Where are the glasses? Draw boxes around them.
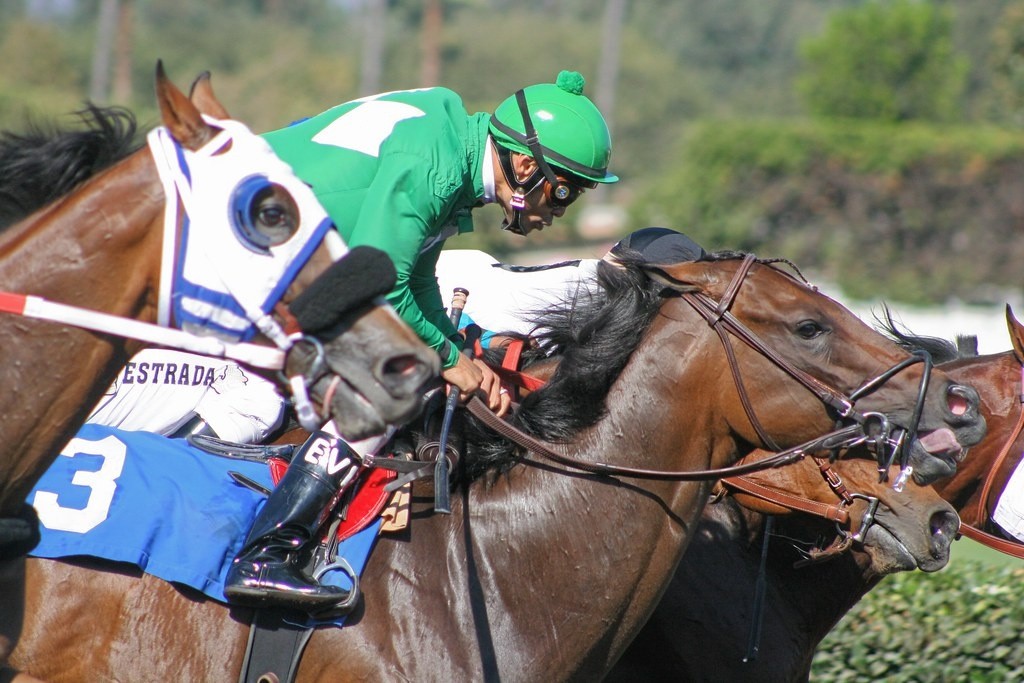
[514,89,584,209]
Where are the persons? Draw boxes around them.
[222,71,618,609]
[436,227,705,355]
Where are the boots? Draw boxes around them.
[222,429,351,616]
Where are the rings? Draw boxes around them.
[500,388,508,395]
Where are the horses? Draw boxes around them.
[0,55,1023,683]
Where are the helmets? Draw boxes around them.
[488,71,619,184]
